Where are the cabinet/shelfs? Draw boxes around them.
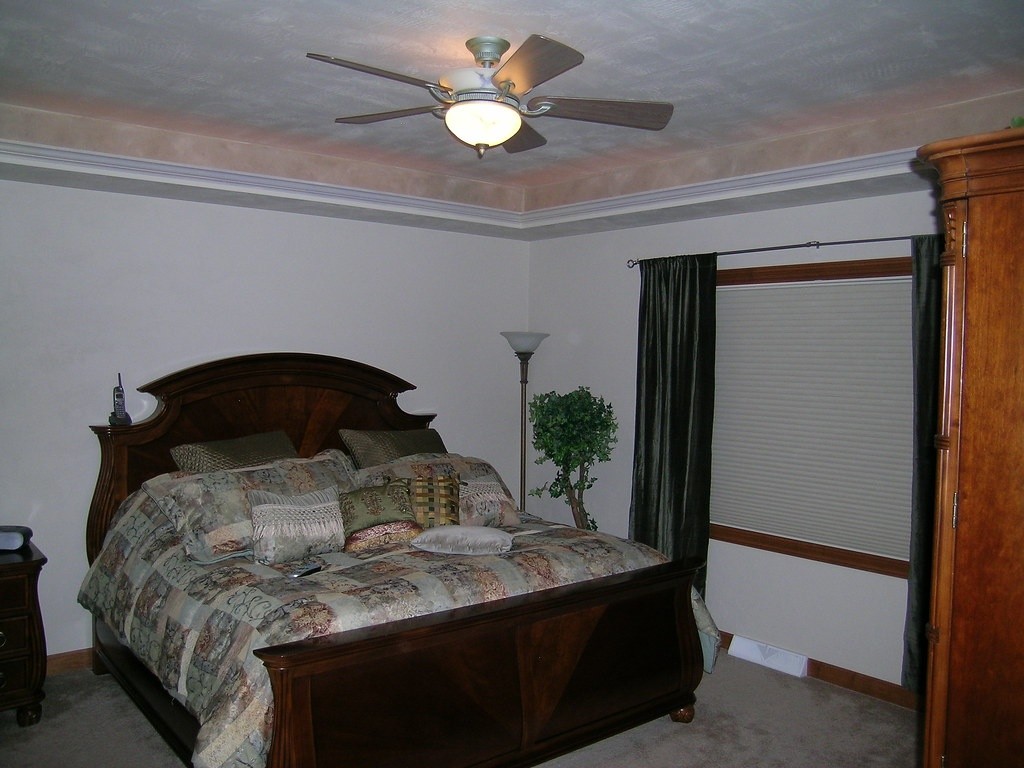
[0,527,47,728]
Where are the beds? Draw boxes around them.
[86,352,703,768]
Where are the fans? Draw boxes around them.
[306,35,673,160]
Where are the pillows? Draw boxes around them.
[350,453,523,528]
[170,429,300,476]
[246,485,344,566]
[141,446,362,564]
[338,478,418,539]
[411,526,515,554]
[338,428,449,470]
[409,473,461,530]
[346,520,423,550]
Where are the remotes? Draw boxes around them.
[288,563,322,578]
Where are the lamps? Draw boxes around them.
[499,330,550,510]
[445,88,522,160]
